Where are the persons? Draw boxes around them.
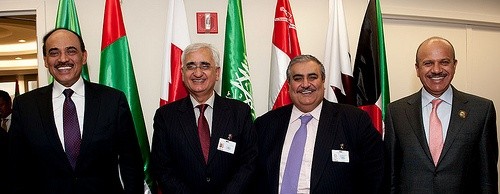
[254,55,383,194]
[7,28,144,194]
[148,43,258,194]
[384,37,499,194]
[0,90,12,133]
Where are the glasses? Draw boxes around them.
[181,64,215,71]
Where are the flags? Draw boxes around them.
[49,0,90,84]
[98,0,150,194]
[323,0,390,135]
[268,0,302,112]
[160,0,191,108]
[221,0,256,121]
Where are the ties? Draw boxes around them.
[429,100,444,166]
[195,103,210,165]
[280,114,313,194]
[62,89,80,172]
